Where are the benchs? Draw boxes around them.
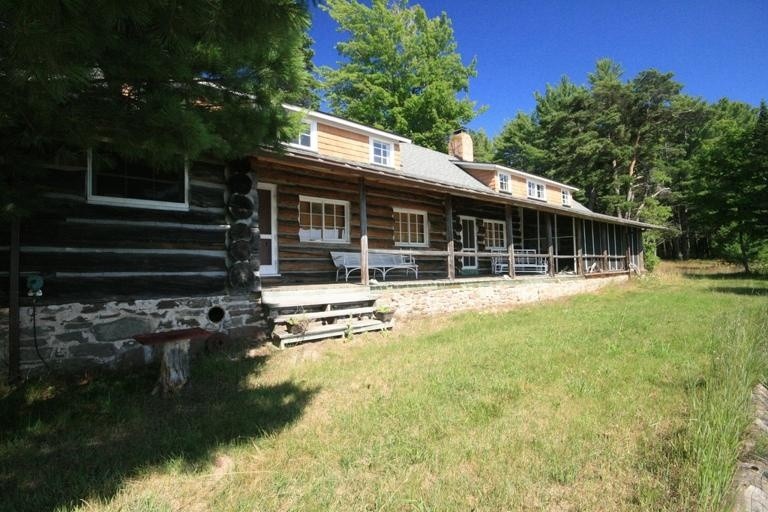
[491,247,548,274]
[330,251,419,283]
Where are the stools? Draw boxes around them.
[132,327,212,400]
